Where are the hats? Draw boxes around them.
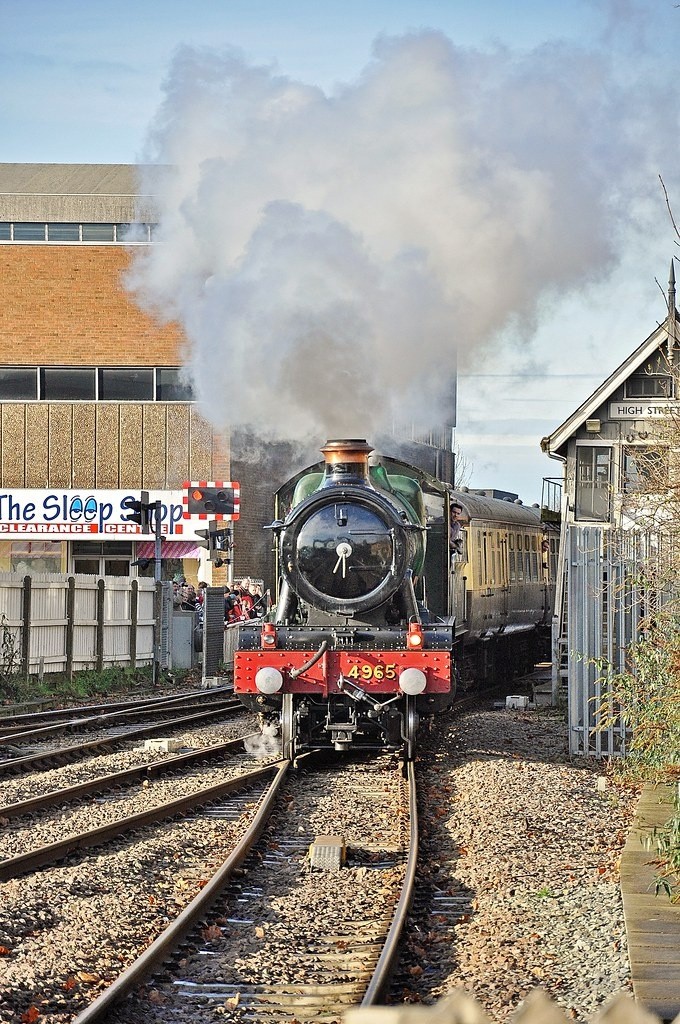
[222,585,230,593]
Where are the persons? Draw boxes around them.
[222,579,273,625]
[172,577,210,622]
[541,540,549,568]
[436,503,463,567]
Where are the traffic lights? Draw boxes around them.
[131,560,149,571]
[187,487,234,514]
[207,558,223,569]
[195,528,208,548]
[126,500,144,526]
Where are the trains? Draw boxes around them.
[231,437,568,763]
